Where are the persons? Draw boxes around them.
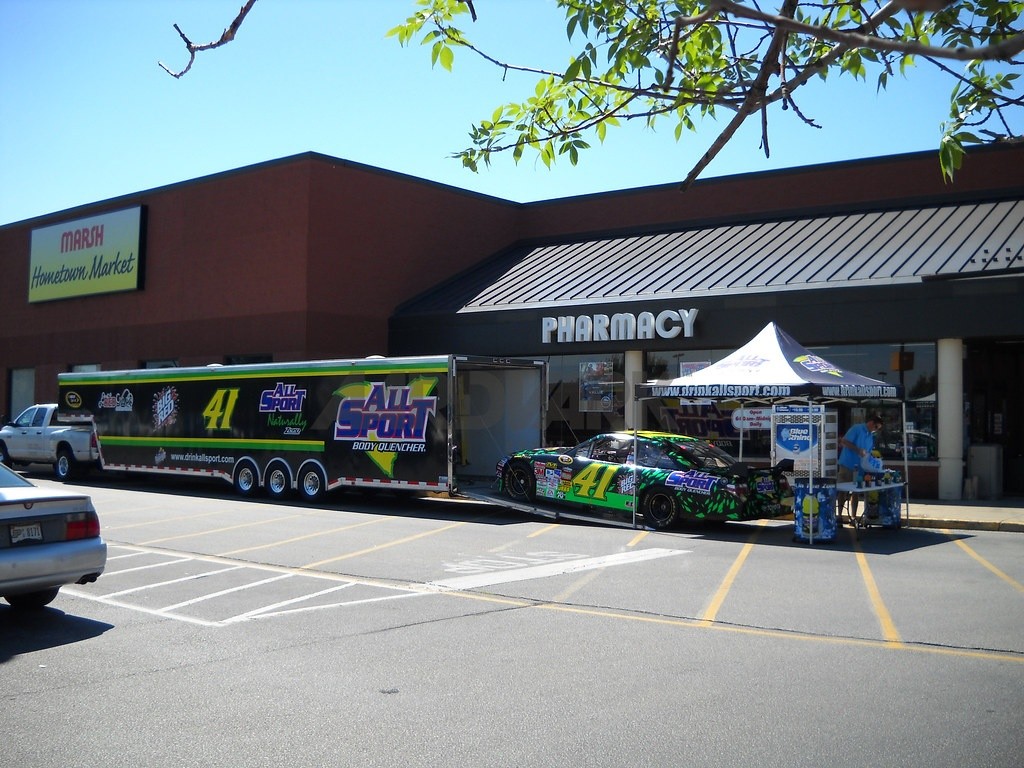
[836,416,883,527]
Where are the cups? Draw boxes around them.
[855,476,900,489]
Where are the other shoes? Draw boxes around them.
[849,519,861,526]
[836,519,842,528]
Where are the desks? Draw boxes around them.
[829,479,905,541]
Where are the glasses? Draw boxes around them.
[874,423,878,429]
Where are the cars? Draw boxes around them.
[497,427,795,532]
[0,464,108,609]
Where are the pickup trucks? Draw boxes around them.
[0,402,102,480]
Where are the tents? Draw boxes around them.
[632,322,909,543]
[908,393,935,408]
